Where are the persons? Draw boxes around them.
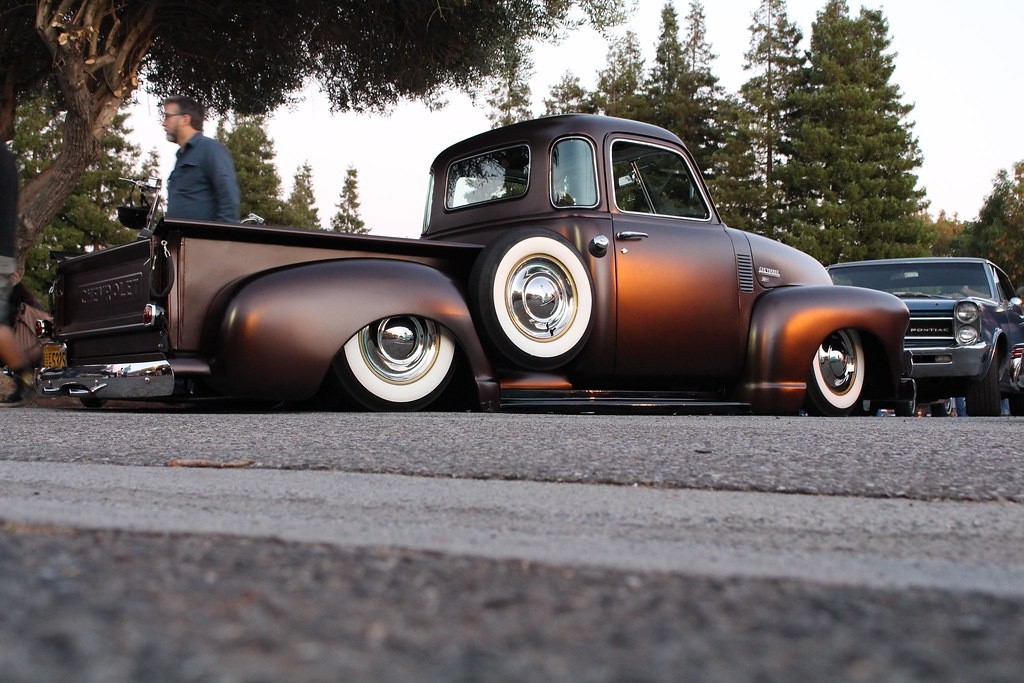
[877,396,1010,417]
[161,96,242,224]
[0,256,45,408]
[117,365,131,379]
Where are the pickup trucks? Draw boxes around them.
[31,113,919,417]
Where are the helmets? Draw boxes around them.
[116,202,153,229]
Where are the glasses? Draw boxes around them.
[164,113,185,119]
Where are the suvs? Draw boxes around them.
[826,256,1024,417]
